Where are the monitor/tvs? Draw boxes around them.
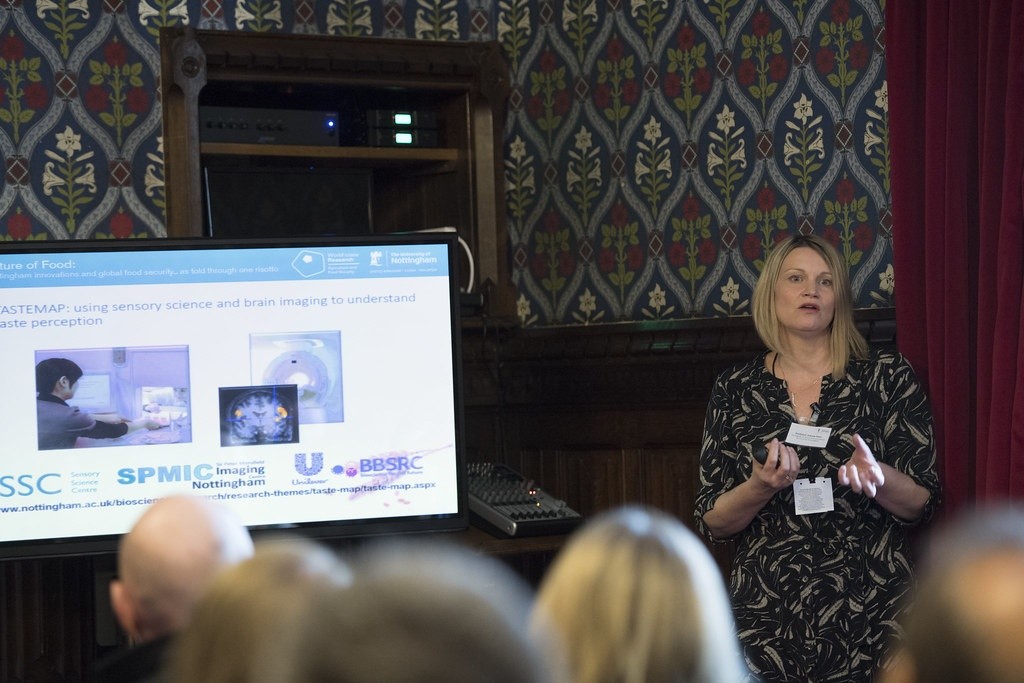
[0,230,466,561]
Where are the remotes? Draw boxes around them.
[752,442,780,469]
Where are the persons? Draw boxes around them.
[879,514,1024,682]
[695,235,942,682]
[35,357,164,452]
[533,506,741,683]
[109,496,552,683]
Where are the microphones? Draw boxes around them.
[810,402,822,413]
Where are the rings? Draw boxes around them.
[785,474,794,482]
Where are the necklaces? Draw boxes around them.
[778,354,832,407]
